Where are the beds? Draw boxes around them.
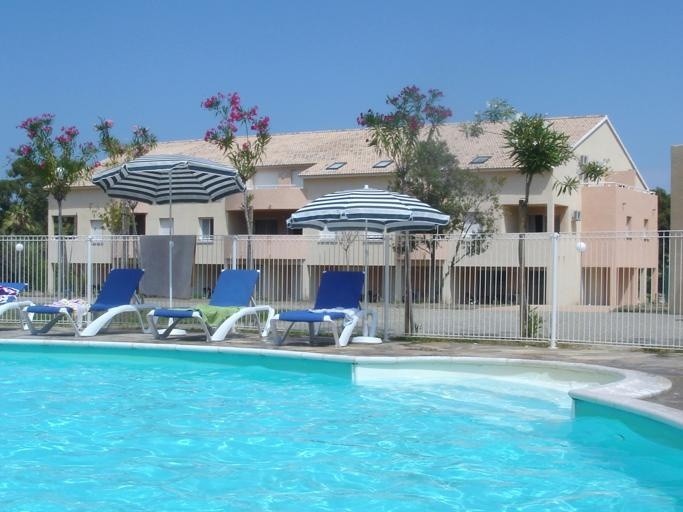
[283,185,451,337]
[91,153,246,307]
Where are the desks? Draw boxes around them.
[573,240,586,306]
[15,242,22,284]
